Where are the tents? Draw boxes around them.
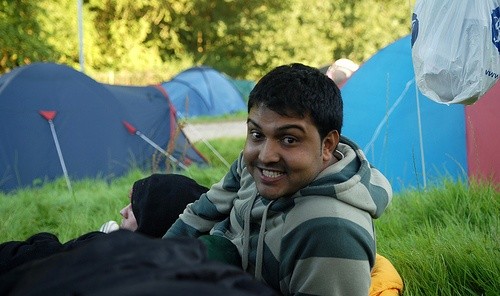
[0,63,245,193]
[340,36,500,197]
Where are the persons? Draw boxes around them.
[120,173,209,296]
[162,63,393,296]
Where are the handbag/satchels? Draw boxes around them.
[411,0,500,106]
